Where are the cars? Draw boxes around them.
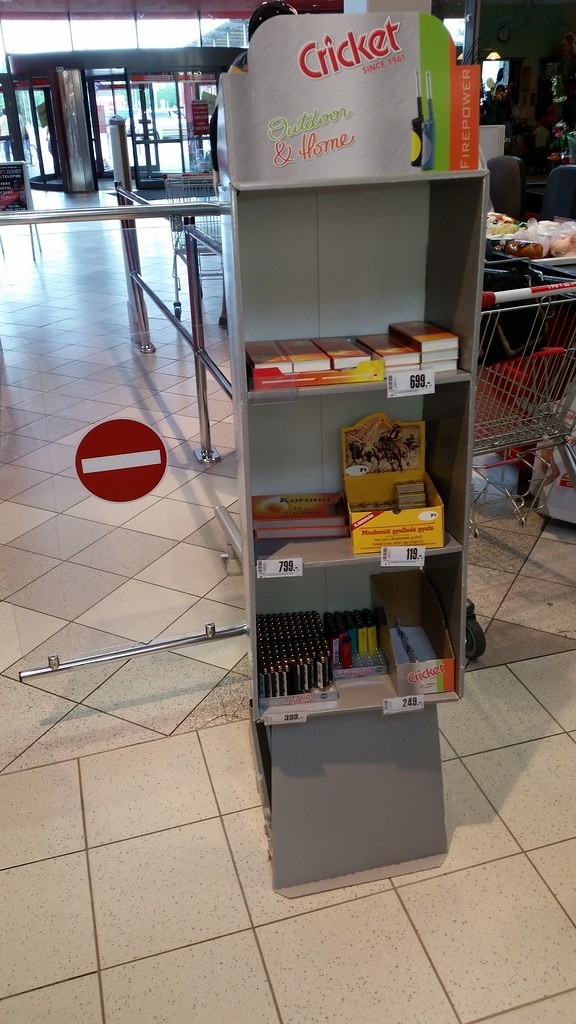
[123,105,211,147]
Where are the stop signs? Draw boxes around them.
[73,419,168,503]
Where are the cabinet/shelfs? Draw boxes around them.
[214,12,490,729]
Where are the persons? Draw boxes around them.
[496,85,513,139]
[0,107,32,162]
[531,103,558,170]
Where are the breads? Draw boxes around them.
[550,234,576,259]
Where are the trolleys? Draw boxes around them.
[471,281,576,538]
[162,172,223,321]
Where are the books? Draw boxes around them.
[245,321,459,377]
[251,493,349,539]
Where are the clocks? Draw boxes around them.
[496,26,511,43]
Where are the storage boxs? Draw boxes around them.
[251,492,345,528]
[332,650,388,679]
[368,570,456,697]
[258,681,340,708]
[256,527,349,539]
[340,413,446,555]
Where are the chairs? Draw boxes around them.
[540,164,576,223]
[486,154,527,222]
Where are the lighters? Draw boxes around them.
[256,608,377,698]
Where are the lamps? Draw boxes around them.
[486,50,501,60]
